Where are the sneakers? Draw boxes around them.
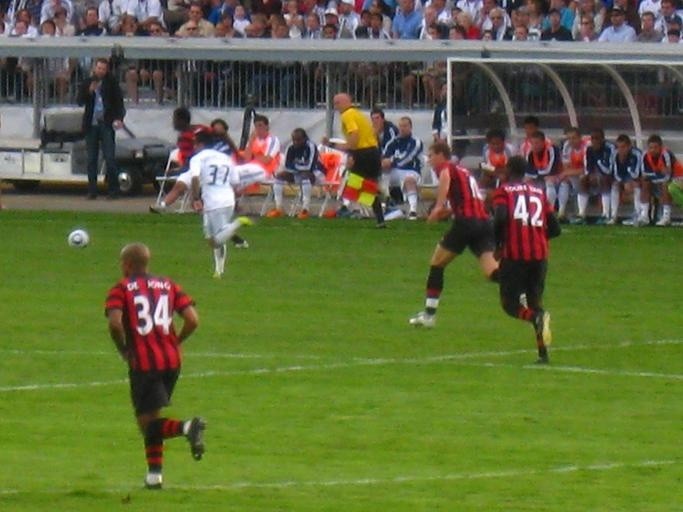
[529,358,550,365]
[408,312,435,328]
[142,480,161,489]
[534,311,551,348]
[299,210,307,218]
[211,272,220,279]
[558,215,671,226]
[264,208,281,217]
[106,191,119,198]
[406,211,416,221]
[185,417,204,461]
[83,192,96,199]
[148,201,165,215]
[236,215,251,227]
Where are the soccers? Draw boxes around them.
[66,229,90,247]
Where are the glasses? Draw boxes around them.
[150,29,158,33]
[489,16,500,20]
[580,21,589,24]
[185,27,197,30]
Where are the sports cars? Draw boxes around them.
[0,109,176,195]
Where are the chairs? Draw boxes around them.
[269,153,306,218]
[457,154,483,172]
[237,151,278,220]
[304,153,344,218]
[151,147,192,214]
[414,156,455,218]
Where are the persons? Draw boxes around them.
[408,142,528,328]
[0,0,682,121]
[104,242,206,489]
[77,58,126,201]
[321,93,386,227]
[484,155,560,365]
[151,109,281,215]
[265,108,682,225]
[188,131,253,280]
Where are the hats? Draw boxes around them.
[323,8,336,16]
[547,9,559,18]
[607,5,622,15]
[665,15,680,24]
[340,0,355,6]
